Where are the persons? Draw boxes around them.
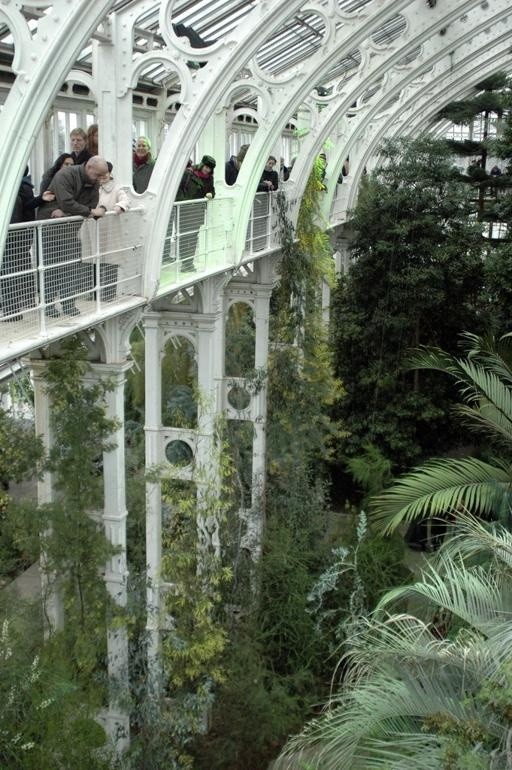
[132,136,155,194]
[226,144,251,185]
[0,124,128,322]
[337,156,350,184]
[162,155,216,273]
[319,154,328,179]
[253,156,289,254]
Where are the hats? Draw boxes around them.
[199,155,216,174]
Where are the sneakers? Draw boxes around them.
[45,306,81,317]
